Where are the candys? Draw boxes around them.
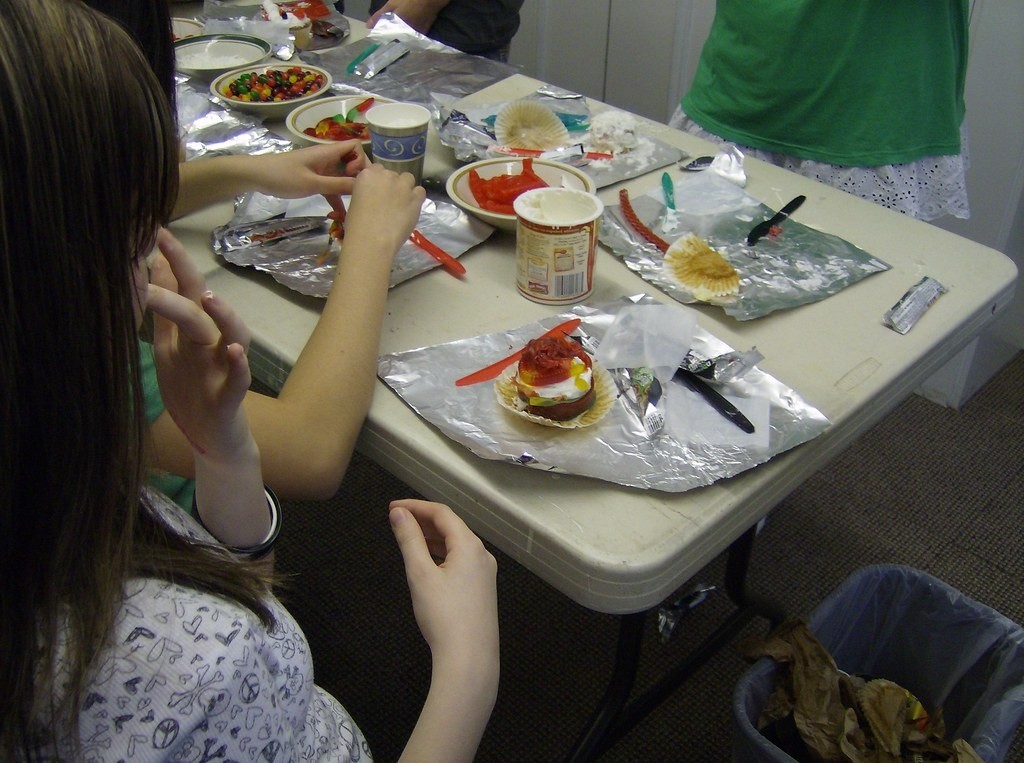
[304,97,376,142]
[222,66,324,103]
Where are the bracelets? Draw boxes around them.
[190,480,283,562]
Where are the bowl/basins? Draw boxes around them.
[446,157,597,234]
[171,17,205,41]
[174,33,272,81]
[285,95,402,156]
[210,63,333,121]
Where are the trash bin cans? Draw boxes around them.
[731,563,1024,763]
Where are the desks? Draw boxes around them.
[150,13,1018,763]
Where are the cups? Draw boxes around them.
[513,187,604,306]
[368,102,431,187]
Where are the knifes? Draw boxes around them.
[747,195,807,246]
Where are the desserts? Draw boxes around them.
[270,7,313,49]
[515,336,598,421]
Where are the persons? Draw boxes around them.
[368,0,525,65]
[667,0,971,534]
[82,0,426,526]
[0,0,499,763]
